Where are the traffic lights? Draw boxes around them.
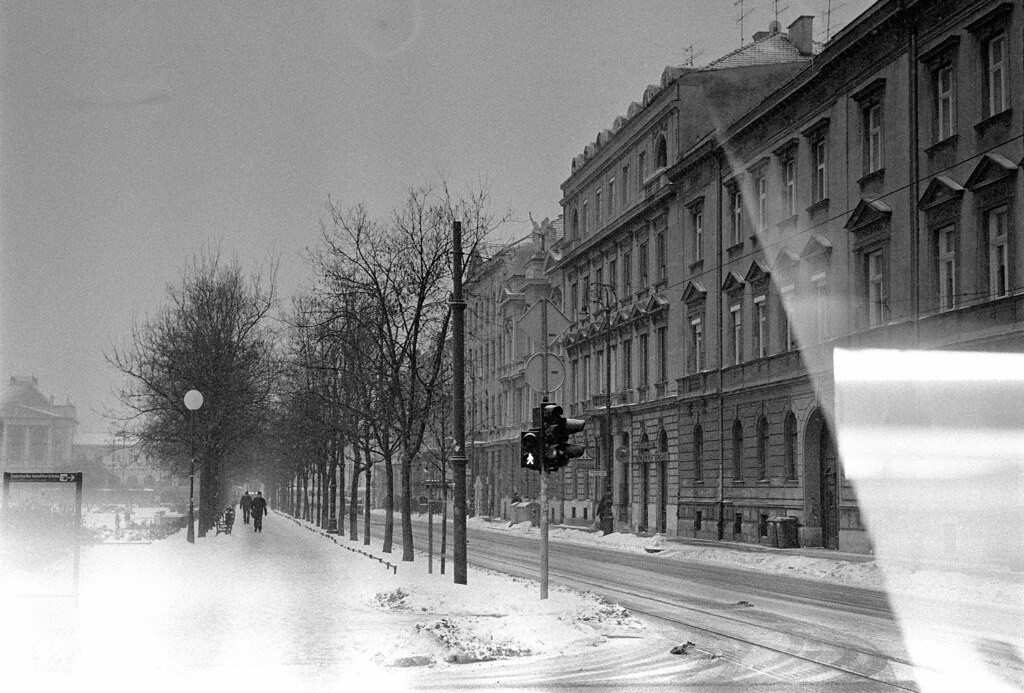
[562,418,586,465]
[520,431,540,470]
[541,402,565,466]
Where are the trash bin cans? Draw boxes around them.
[766,516,800,549]
[419,503,429,516]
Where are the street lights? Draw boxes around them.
[579,282,613,536]
[183,390,204,544]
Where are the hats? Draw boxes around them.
[245,491,248,494]
[257,492,261,495]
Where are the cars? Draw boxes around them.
[343,496,363,514]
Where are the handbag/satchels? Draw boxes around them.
[251,509,257,517]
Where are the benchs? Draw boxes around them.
[214,511,232,536]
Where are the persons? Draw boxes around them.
[419,494,427,503]
[251,491,267,532]
[512,492,523,503]
[540,219,548,251]
[240,491,251,524]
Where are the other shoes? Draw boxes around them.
[255,527,256,531]
[259,528,262,532]
